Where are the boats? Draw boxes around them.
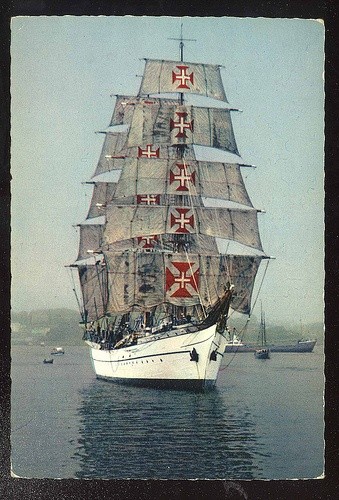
[44,359,53,363]
[51,348,65,355]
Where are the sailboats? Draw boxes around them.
[63,34,277,392]
[220,299,319,353]
[255,299,270,360]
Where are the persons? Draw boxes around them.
[86,322,124,350]
[157,313,191,333]
